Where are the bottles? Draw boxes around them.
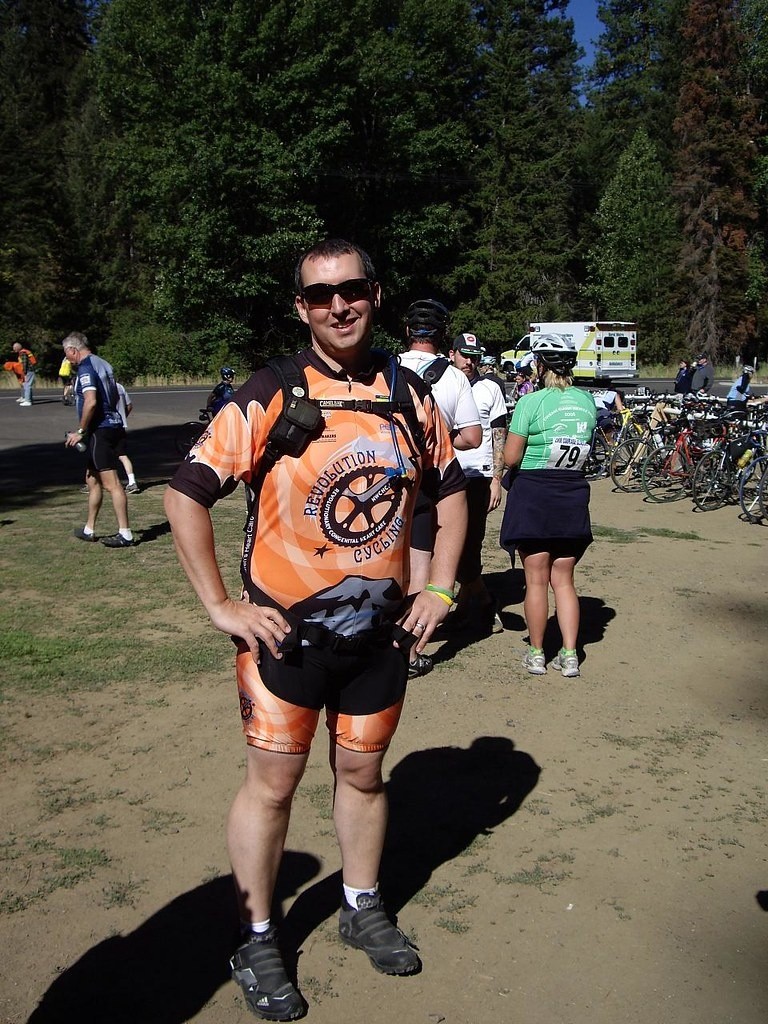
[65,430,86,452]
[654,433,665,453]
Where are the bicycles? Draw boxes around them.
[175,408,213,457]
[63,377,76,406]
[584,391,768,524]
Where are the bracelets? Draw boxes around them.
[424,582,460,612]
[76,428,86,436]
[493,466,503,481]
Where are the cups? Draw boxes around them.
[737,449,753,467]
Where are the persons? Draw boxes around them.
[726,366,755,420]
[165,240,468,1021]
[391,298,483,678]
[504,333,598,677]
[4,342,37,406]
[478,355,534,403]
[61,332,135,547]
[207,368,236,419]
[80,382,140,494]
[592,389,624,475]
[58,357,77,403]
[447,333,507,634]
[675,354,715,396]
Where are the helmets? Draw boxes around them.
[742,365,756,375]
[403,299,451,338]
[531,332,580,375]
[220,367,237,376]
[480,355,498,367]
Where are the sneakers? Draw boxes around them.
[100,533,136,548]
[227,926,309,1023]
[408,652,434,679]
[521,651,548,675]
[71,526,97,542]
[551,648,581,678]
[338,895,418,973]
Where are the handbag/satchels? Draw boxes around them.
[705,418,729,437]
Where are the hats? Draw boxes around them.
[452,333,484,355]
[695,352,708,360]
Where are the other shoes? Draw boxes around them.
[468,596,503,632]
[59,396,72,406]
[124,483,141,494]
[81,481,89,494]
[15,397,32,406]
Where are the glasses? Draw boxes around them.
[298,278,373,304]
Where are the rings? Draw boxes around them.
[416,623,425,631]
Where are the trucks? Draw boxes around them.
[500,321,639,388]
